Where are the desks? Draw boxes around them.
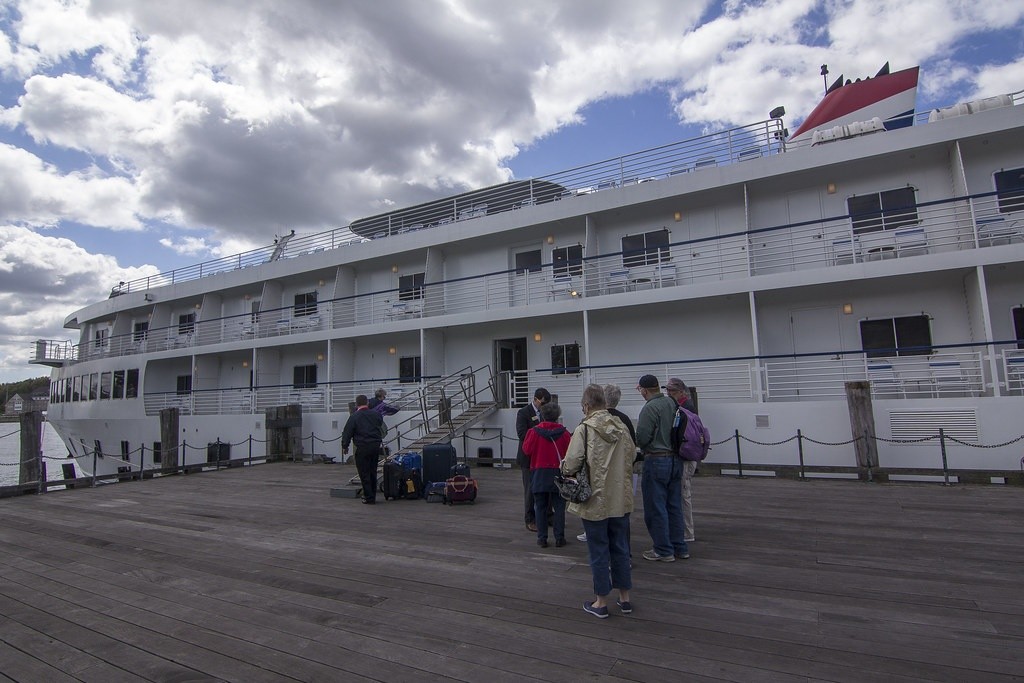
[903,379,934,398]
[631,279,651,292]
[867,246,896,262]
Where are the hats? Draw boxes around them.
[635,374,659,389]
[661,377,686,391]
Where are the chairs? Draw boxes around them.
[207,144,762,279]
[384,384,403,407]
[174,331,192,349]
[603,261,677,295]
[832,226,931,266]
[278,315,320,336]
[546,273,573,302]
[383,300,406,322]
[122,338,143,356]
[976,217,1024,246]
[867,355,974,399]
[1009,351,1024,396]
[289,390,323,413]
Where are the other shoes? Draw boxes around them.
[555,538,566,547]
[583,601,609,619]
[362,499,375,505]
[617,597,632,613]
[537,538,548,548]
[684,536,696,542]
[361,495,367,500]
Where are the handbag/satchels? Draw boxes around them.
[553,422,591,504]
[424,480,447,503]
[378,420,388,439]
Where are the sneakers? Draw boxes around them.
[576,532,587,542]
[674,547,691,559]
[640,549,676,562]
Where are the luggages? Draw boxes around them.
[395,451,422,472]
[381,439,403,501]
[422,442,458,482]
[451,462,471,477]
[444,474,478,505]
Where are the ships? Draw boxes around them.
[28,87,1024,483]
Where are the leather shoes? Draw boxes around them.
[526,521,538,532]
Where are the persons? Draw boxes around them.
[576,385,634,569]
[522,403,570,547]
[659,378,698,543]
[516,388,553,530]
[559,384,633,619]
[634,375,690,563]
[342,388,402,503]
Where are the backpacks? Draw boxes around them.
[401,467,424,500]
[667,394,711,461]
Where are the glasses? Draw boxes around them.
[383,396,386,398]
[534,396,545,406]
